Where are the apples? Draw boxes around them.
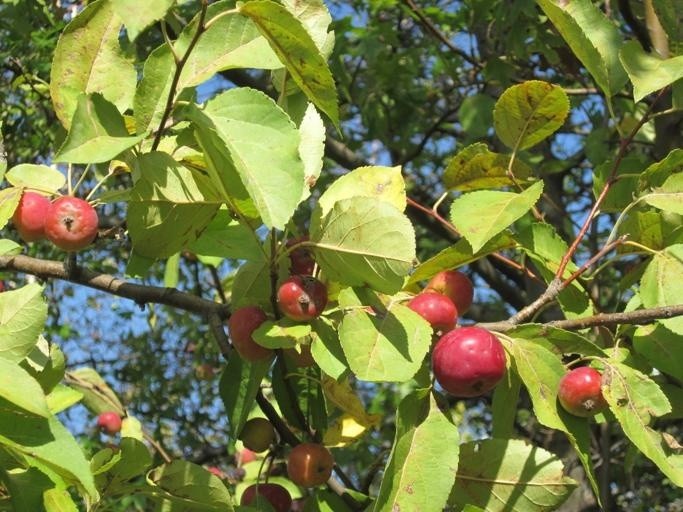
[11,191,607,512]
